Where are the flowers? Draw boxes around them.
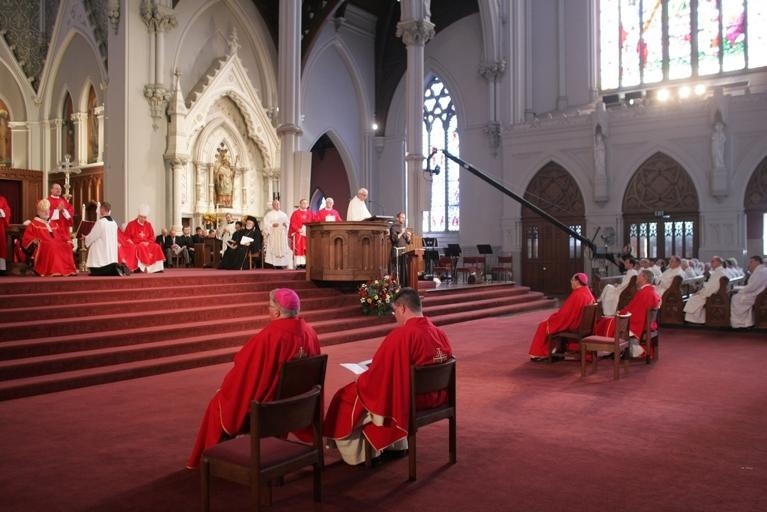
[356,271,402,317]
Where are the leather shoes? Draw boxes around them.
[371,450,408,465]
[168,264,172,268]
[115,263,130,276]
[603,352,623,360]
[531,357,549,363]
[186,264,191,268]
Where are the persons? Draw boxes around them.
[617,245,635,274]
[287,199,319,268]
[389,213,411,288]
[323,286,453,466]
[595,255,767,330]
[347,187,372,221]
[215,156,236,208]
[317,197,341,221]
[262,199,290,267]
[0,183,262,276]
[528,272,595,361]
[183,289,325,471]
[595,270,659,360]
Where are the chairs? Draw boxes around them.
[624,309,659,365]
[592,275,767,333]
[200,385,321,510]
[428,255,514,286]
[580,313,632,381]
[548,303,601,364]
[364,355,457,480]
[266,355,329,486]
[157,238,265,271]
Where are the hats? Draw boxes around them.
[137,205,150,217]
[275,289,301,309]
[579,273,588,284]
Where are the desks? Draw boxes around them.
[5,223,37,274]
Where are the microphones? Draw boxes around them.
[368,200,385,214]
